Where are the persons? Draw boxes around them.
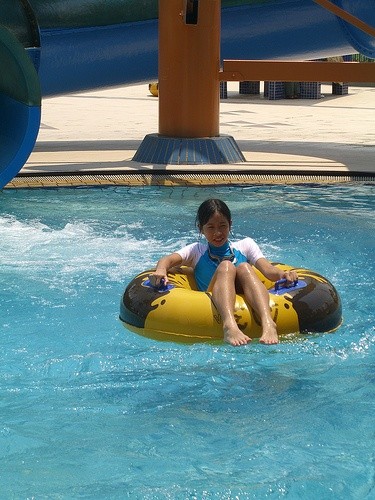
[148,198,298,347]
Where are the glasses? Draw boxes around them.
[208,242,235,264]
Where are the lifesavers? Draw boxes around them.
[91,366,317,421]
[118,260,345,347]
